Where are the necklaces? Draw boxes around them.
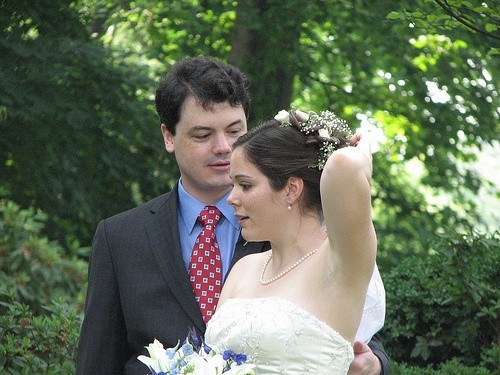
[260,249,320,283]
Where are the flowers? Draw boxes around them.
[136,326,257,375]
[274,107,355,172]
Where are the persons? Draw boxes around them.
[196,110,388,375]
[69,54,397,375]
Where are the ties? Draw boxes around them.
[188,206,224,326]
